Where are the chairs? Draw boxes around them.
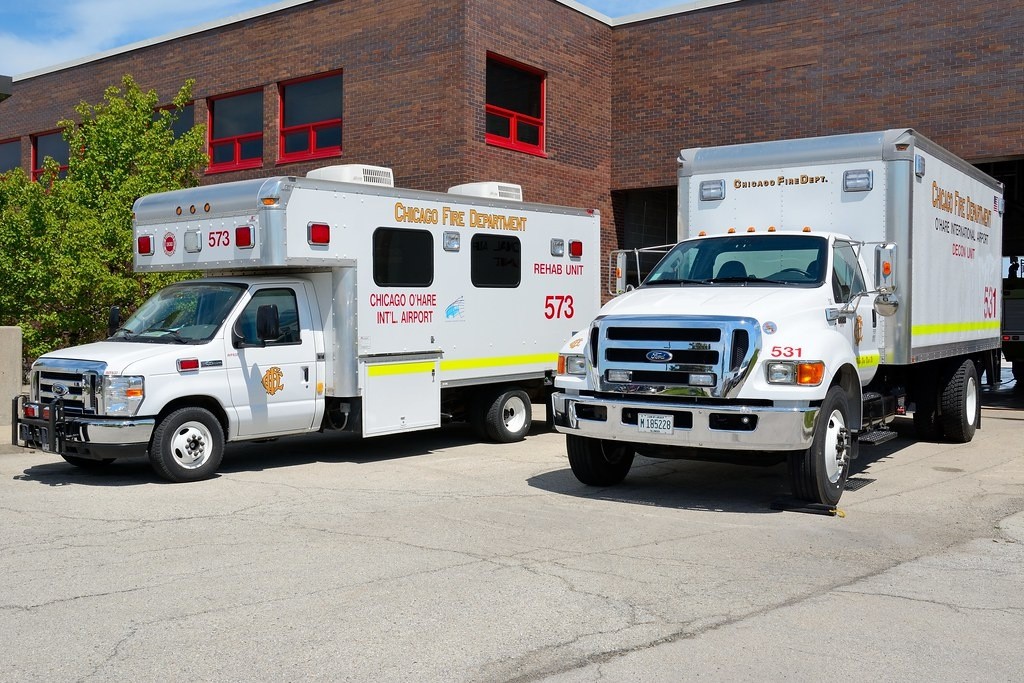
[256,305,280,344]
[807,260,843,302]
[715,260,747,280]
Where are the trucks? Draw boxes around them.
[551,127,1002,510]
[11,164,603,485]
